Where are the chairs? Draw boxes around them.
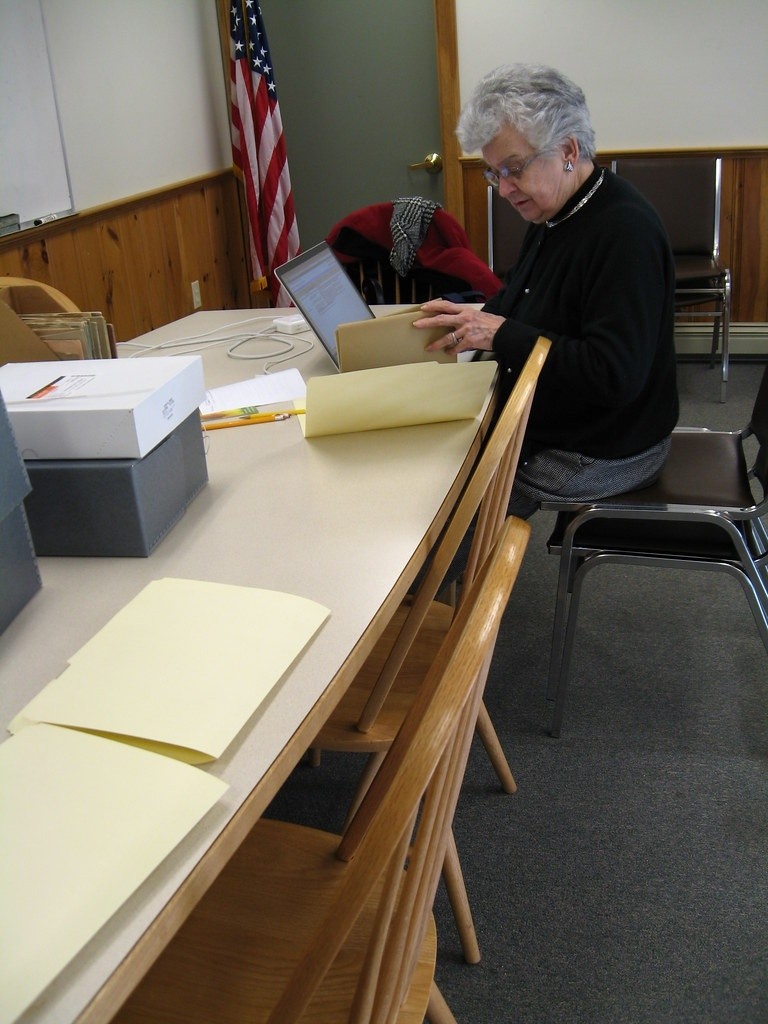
[487,184,529,287]
[111,514,531,1024]
[306,336,554,966]
[609,156,731,403]
[322,199,465,310]
[538,368,768,740]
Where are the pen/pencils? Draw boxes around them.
[199,406,306,430]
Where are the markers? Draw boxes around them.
[34,214,57,226]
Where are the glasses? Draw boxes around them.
[482,150,545,187]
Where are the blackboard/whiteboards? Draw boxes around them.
[0,0,235,257]
[433,0,768,173]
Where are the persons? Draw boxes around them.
[405,60,683,600]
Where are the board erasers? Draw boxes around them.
[0,212,22,236]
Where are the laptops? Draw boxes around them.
[274,241,376,372]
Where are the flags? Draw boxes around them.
[228,0,305,310]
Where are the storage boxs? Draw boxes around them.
[0,354,206,460]
[21,406,211,558]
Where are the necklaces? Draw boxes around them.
[543,169,606,229]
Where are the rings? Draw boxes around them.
[451,332,459,346]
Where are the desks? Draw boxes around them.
[0,302,511,1024]
